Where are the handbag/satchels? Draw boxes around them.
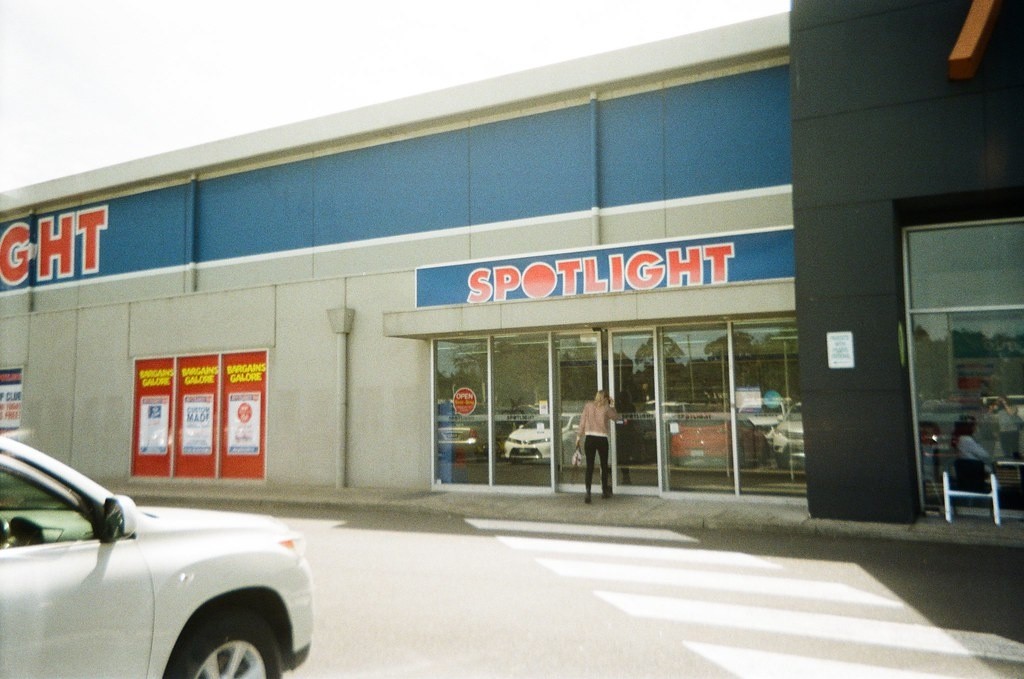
[571,445,582,467]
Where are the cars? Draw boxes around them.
[739,396,795,441]
[773,401,805,471]
[503,413,609,468]
[631,400,695,443]
[666,412,770,469]
[984,395,1024,438]
[0,435,313,679]
[435,423,513,463]
[916,398,984,457]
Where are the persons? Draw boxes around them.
[988,393,1020,460]
[946,416,993,494]
[619,390,646,464]
[576,390,622,503]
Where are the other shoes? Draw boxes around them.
[584,495,591,503]
[602,491,611,498]
